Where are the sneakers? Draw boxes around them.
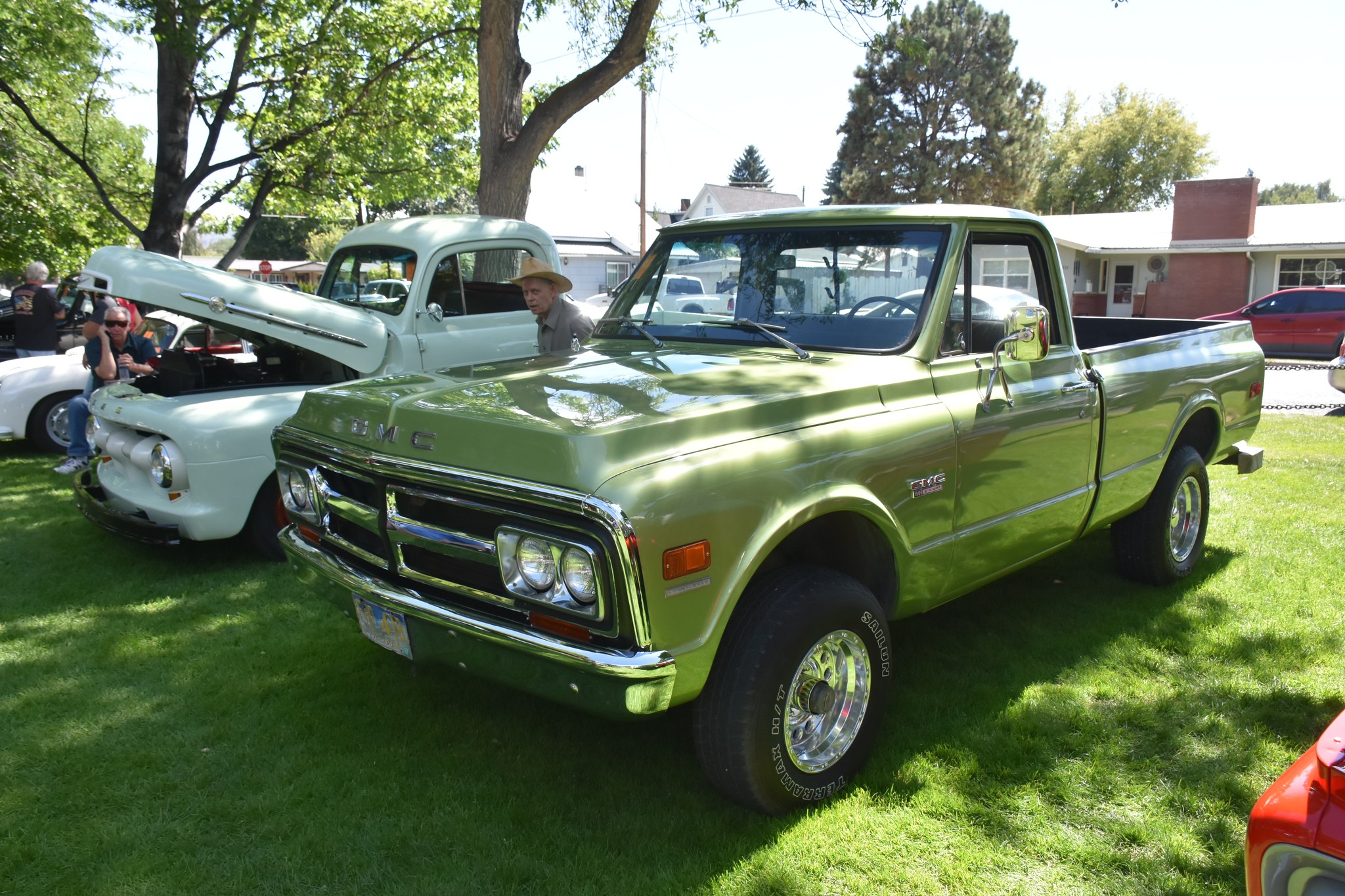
[52,457,92,474]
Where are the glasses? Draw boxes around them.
[103,319,128,329]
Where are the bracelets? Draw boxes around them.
[150,368,155,374]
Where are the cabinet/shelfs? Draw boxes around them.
[1073,292,1122,317]
[1132,293,1146,318]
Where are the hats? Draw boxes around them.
[504,257,573,294]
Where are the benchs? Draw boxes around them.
[773,314,1005,351]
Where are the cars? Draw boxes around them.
[0,269,163,362]
[832,284,1041,321]
[1193,286,1345,360]
[267,278,412,305]
[73,212,665,563]
[0,309,259,463]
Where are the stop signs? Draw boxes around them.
[259,261,272,275]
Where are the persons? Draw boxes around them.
[83,290,118,340]
[505,255,594,355]
[114,296,142,333]
[11,262,64,358]
[53,307,158,474]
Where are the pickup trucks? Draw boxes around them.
[269,202,1268,824]
[584,274,737,318]
